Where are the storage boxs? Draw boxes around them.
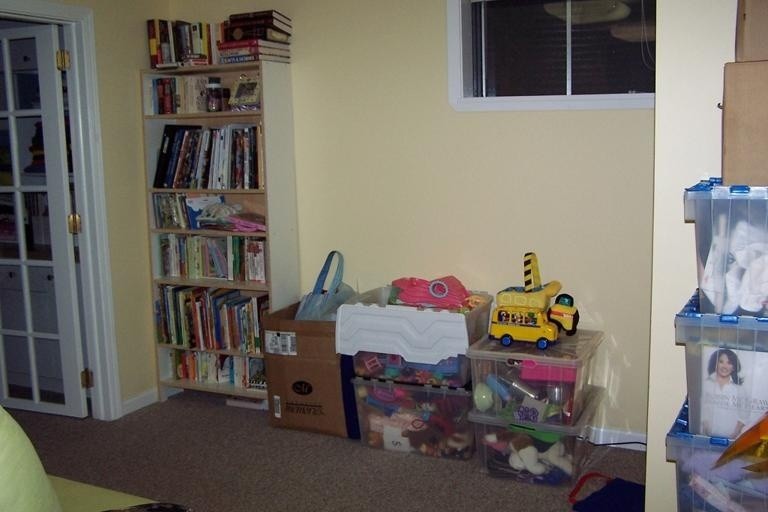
[468,324,604,426]
[261,291,355,436]
[345,287,494,390]
[722,59,767,187]
[345,375,477,461]
[670,290,767,447]
[679,174,768,320]
[663,395,767,512]
[734,0,767,63]
[484,383,609,486]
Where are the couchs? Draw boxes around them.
[0,402,160,512]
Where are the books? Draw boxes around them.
[152,282,266,356]
[152,124,265,190]
[169,347,266,392]
[156,234,265,282]
[152,191,264,232]
[146,9,292,67]
[151,76,209,114]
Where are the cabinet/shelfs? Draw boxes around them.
[0,38,88,398]
[139,61,302,404]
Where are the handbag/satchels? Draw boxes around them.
[295,251,353,321]
[569,472,645,512]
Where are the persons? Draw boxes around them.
[701,349,749,439]
